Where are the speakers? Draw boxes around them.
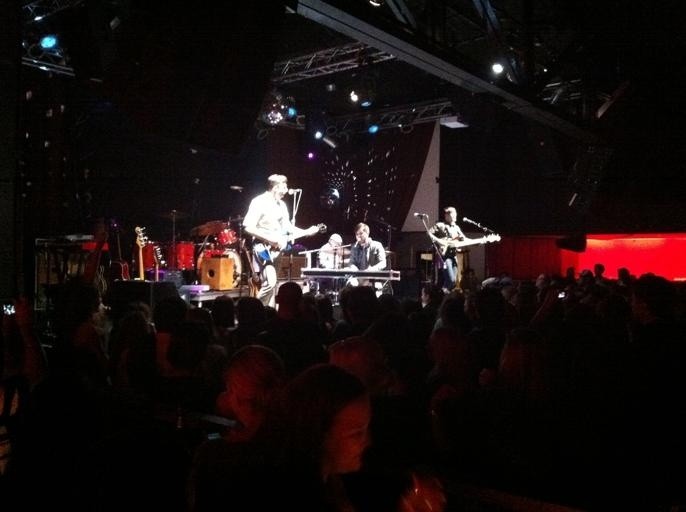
[576,208,614,233]
[112,278,190,329]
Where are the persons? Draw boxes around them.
[316,232,344,268]
[242,173,319,311]
[2,263,686,512]
[346,222,387,298]
[426,205,468,293]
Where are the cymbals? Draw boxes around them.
[190,219,233,244]
[159,209,190,221]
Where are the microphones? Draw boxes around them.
[413,212,427,218]
[462,217,482,228]
[354,237,362,248]
[288,188,301,195]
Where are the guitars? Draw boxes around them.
[429,234,502,259]
[251,223,328,266]
[108,219,131,282]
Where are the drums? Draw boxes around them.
[167,241,198,272]
[212,228,239,248]
[196,246,245,289]
[130,240,156,273]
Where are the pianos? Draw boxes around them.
[301,267,402,282]
